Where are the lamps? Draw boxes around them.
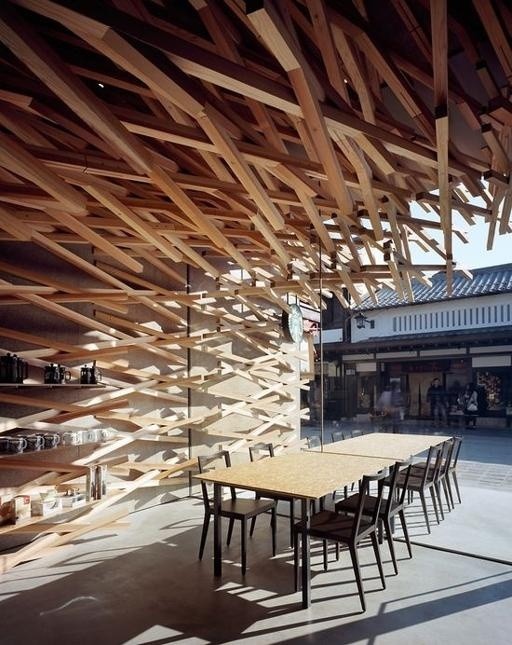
[353,309,375,330]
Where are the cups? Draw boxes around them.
[0,352,103,385]
[0,428,112,455]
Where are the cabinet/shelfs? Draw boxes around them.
[0,380,123,541]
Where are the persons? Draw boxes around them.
[307,376,480,431]
